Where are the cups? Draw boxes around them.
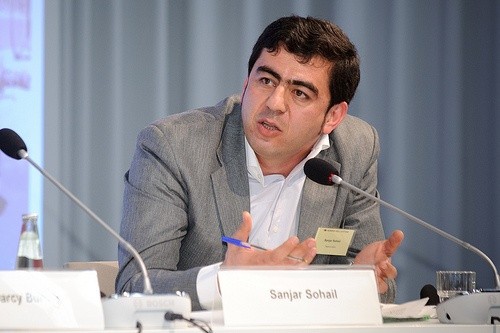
[436,271,477,303]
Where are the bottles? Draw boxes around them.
[16,214,43,271]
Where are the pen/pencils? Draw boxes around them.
[221,236,309,264]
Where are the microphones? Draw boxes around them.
[304,158,500,325]
[0,128,192,330]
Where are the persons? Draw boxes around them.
[113,14,404,313]
